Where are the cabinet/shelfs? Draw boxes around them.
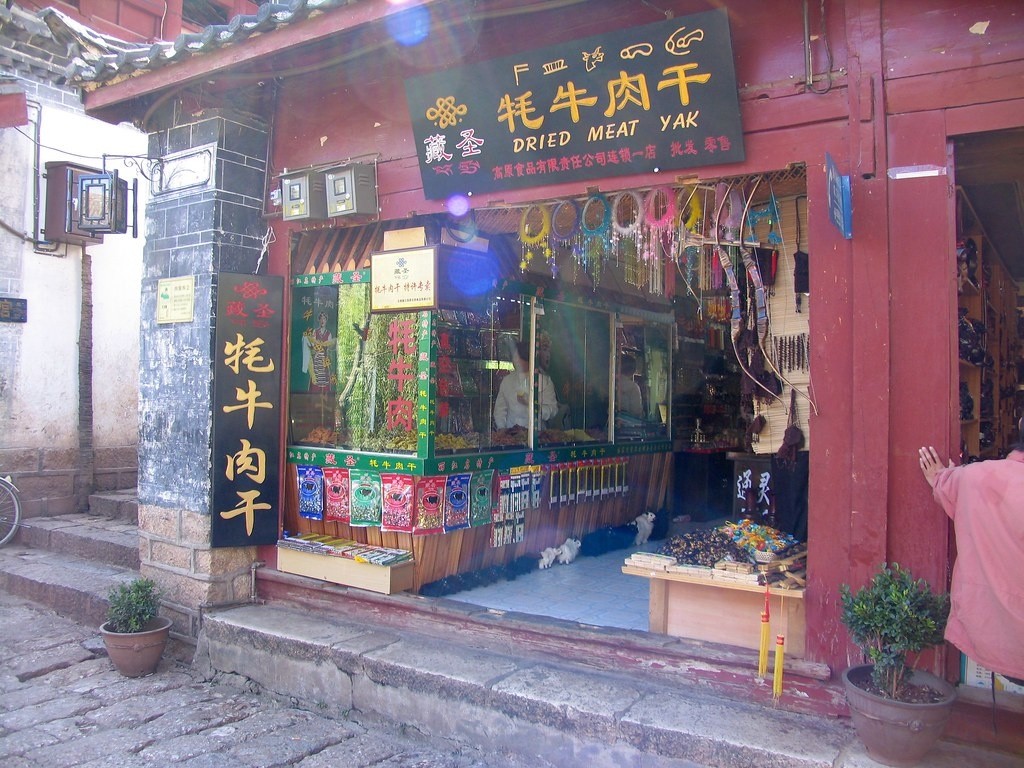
[277,548,417,594]
[953,184,1019,466]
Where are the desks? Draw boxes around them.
[724,450,810,543]
[669,445,744,523]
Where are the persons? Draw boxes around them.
[618,354,644,422]
[494,341,558,430]
[918,410,1024,698]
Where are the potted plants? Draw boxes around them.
[835,559,956,768]
[99,570,175,677]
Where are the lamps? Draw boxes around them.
[887,165,946,179]
[60,155,164,239]
